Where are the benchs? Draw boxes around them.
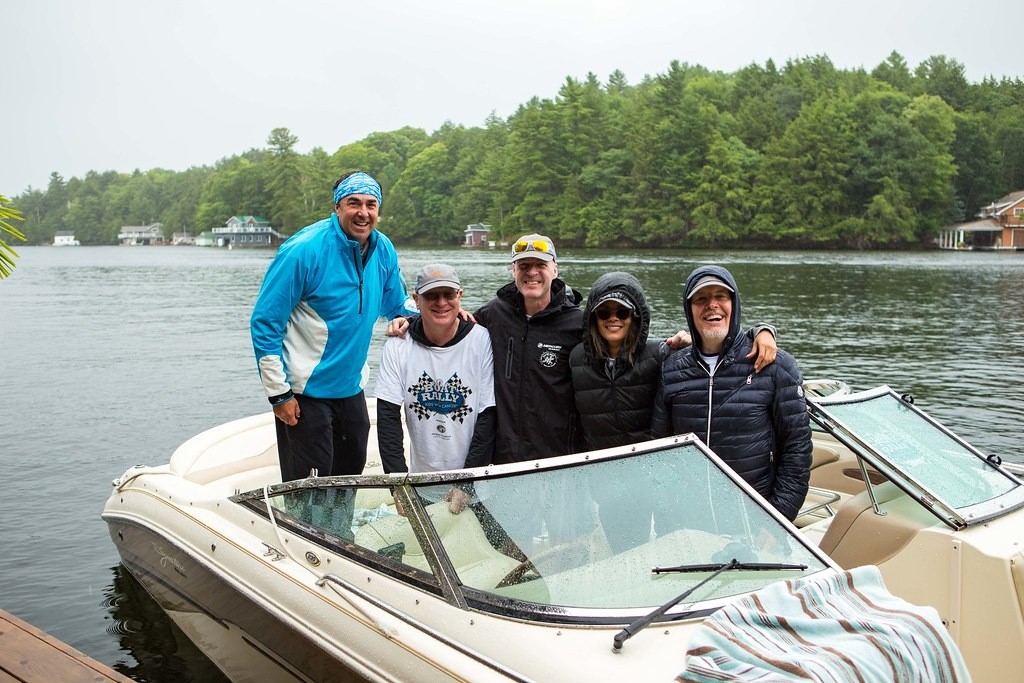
[792,439,890,527]
[169,397,412,515]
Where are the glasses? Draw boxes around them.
[512,240,554,258]
[422,290,459,300]
[594,308,631,320]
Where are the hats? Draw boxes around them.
[591,292,633,312]
[510,233,556,263]
[687,275,734,299]
[414,264,460,294]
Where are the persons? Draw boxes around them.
[652,265,813,557]
[390,232,695,564]
[250,169,478,540]
[375,263,497,515]
[570,270,778,452]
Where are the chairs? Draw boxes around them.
[354,501,535,591]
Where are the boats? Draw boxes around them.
[102,383,1023,683]
